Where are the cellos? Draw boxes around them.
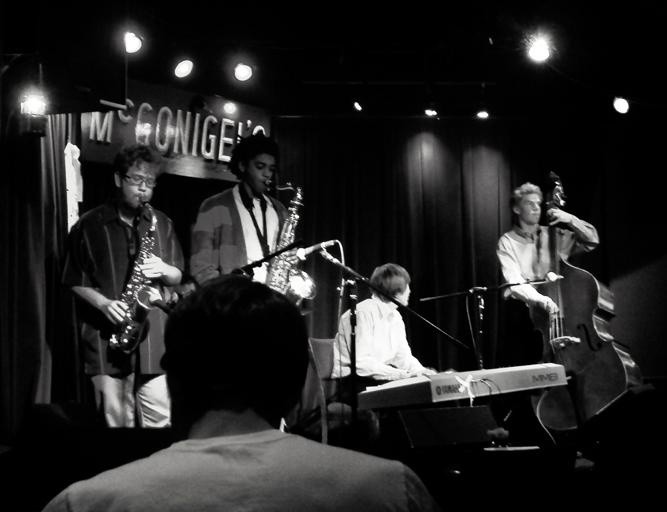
[529,172,644,438]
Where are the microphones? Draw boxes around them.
[148,294,170,317]
[546,271,564,284]
[295,238,335,260]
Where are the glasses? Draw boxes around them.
[124,174,156,188]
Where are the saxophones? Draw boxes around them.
[109,199,167,354]
[264,178,318,308]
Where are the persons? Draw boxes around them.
[42,142,186,433]
[328,261,443,448]
[489,180,601,452]
[41,272,443,511]
[188,131,302,315]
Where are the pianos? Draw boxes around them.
[358,363,569,411]
